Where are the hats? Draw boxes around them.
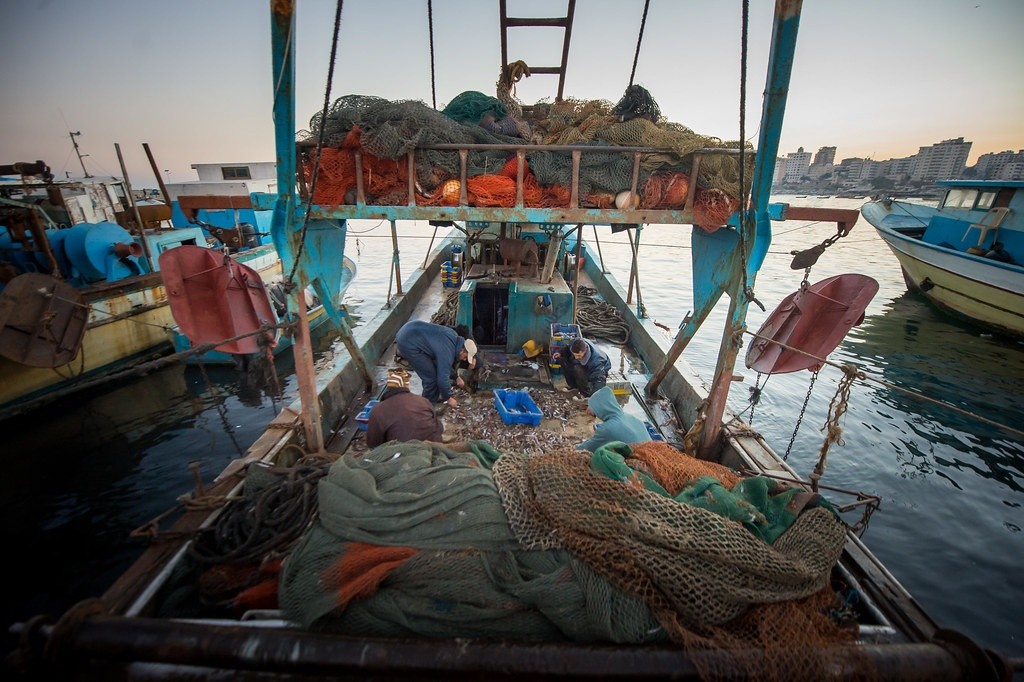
[464,339,477,364]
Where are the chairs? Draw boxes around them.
[961,207,1011,246]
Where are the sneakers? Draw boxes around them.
[562,386,578,392]
[573,393,589,401]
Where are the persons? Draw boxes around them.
[552,337,611,417]
[571,386,652,453]
[396,319,483,409]
[367,387,444,450]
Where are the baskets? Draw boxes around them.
[440,261,461,287]
[355,400,381,431]
[494,388,544,427]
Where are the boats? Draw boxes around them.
[858,178,1024,331]
[0,159,358,420]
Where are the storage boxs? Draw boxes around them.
[440,260,463,288]
[605,370,633,395]
[548,322,584,378]
[355,400,382,431]
[493,389,544,427]
